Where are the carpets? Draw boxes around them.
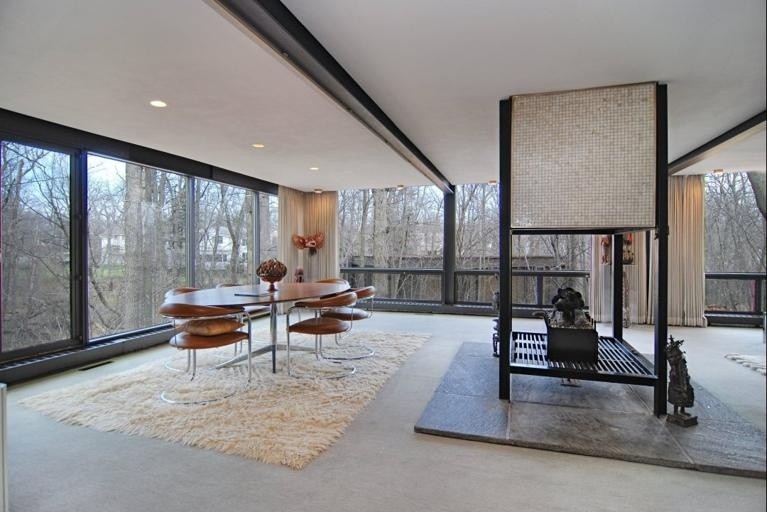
[18,321,432,470]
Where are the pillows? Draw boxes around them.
[176,319,245,336]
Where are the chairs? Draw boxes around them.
[320,286,376,360]
[165,287,243,374]
[216,284,271,354]
[296,277,350,322]
[158,303,252,404]
[286,292,357,379]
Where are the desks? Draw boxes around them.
[165,283,350,373]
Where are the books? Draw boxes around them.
[235,292,272,297]
